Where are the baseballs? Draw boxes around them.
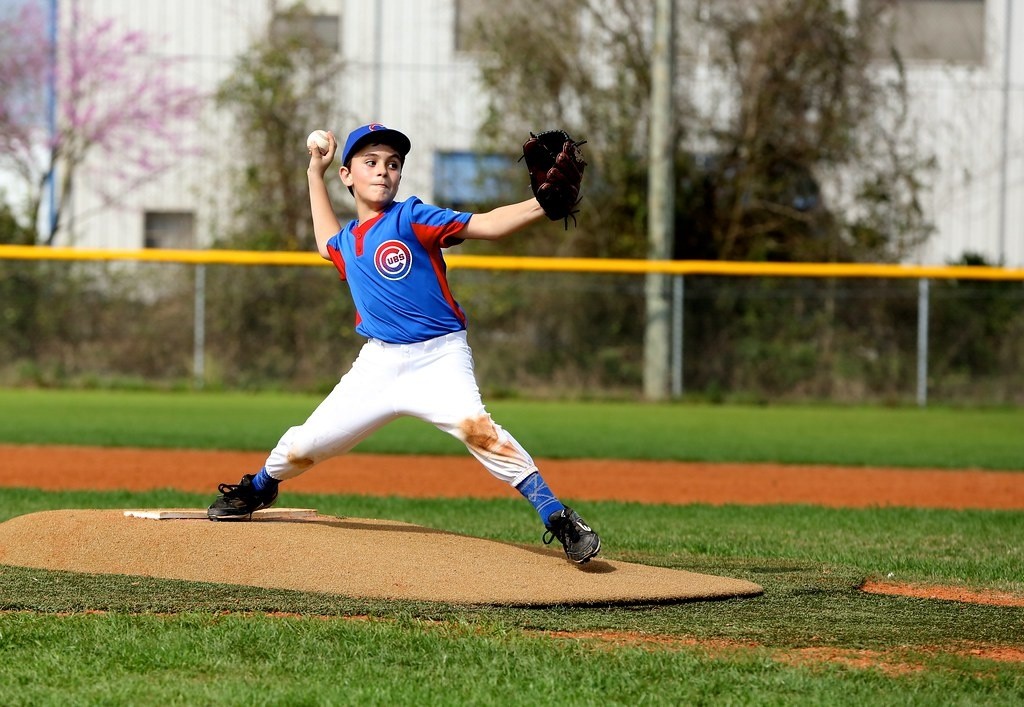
[306,129,330,156]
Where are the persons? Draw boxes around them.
[206,122,603,564]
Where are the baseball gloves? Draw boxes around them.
[516,129,589,232]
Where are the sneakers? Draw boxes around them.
[207,474,279,522]
[543,504,601,564]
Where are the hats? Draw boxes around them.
[341,123,411,198]
